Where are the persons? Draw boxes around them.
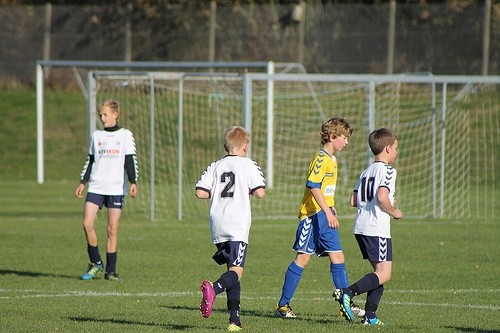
[273,119,367,319]
[76,101,141,280]
[333,128,402,326]
[195,126,266,332]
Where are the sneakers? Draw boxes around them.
[274,303,298,318]
[201,281,216,317]
[226,323,242,331]
[361,315,388,326]
[80,260,104,280]
[339,301,366,318]
[332,288,354,322]
[104,271,120,281]
